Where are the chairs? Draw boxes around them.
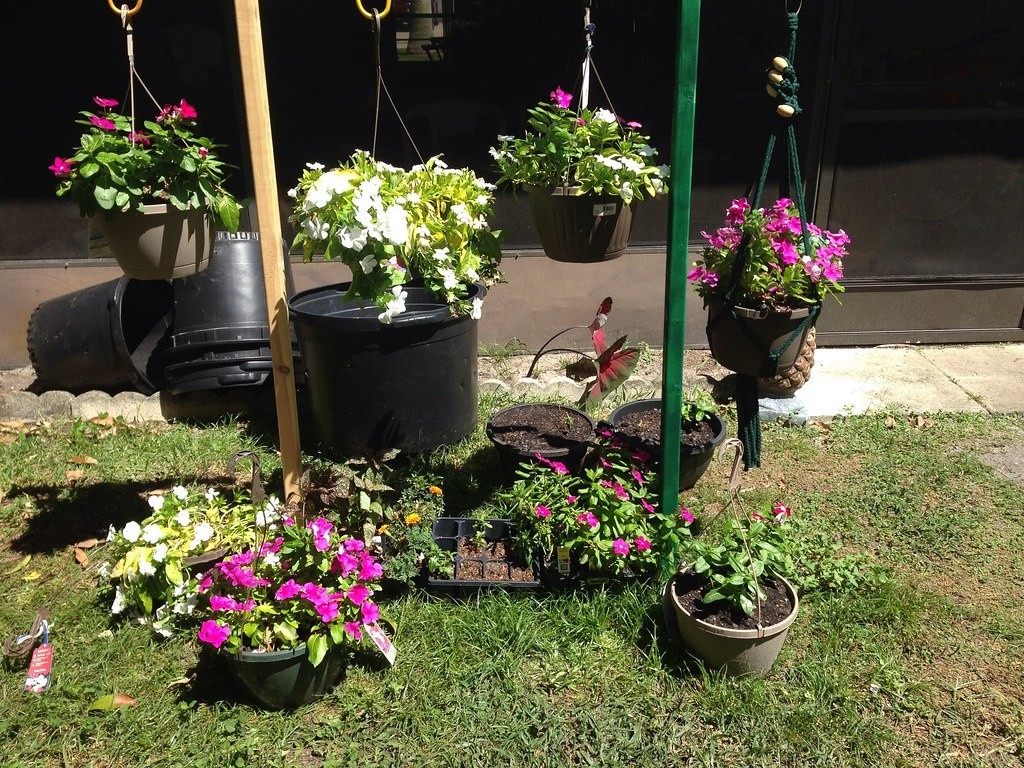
[402,99,507,164]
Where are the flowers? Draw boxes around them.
[488,86,671,203]
[689,196,850,307]
[94,479,287,638]
[198,511,383,668]
[47,96,243,233]
[502,432,693,567]
[693,499,801,615]
[287,150,505,321]
[378,472,445,580]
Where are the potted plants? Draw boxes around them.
[486,400,595,477]
[426,509,542,594]
[607,385,727,494]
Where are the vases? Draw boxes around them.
[159,229,301,435]
[24,273,158,394]
[288,278,487,471]
[101,200,212,281]
[670,561,799,678]
[520,181,633,263]
[180,550,226,626]
[606,572,652,592]
[548,549,581,594]
[206,642,333,711]
[705,298,814,375]
